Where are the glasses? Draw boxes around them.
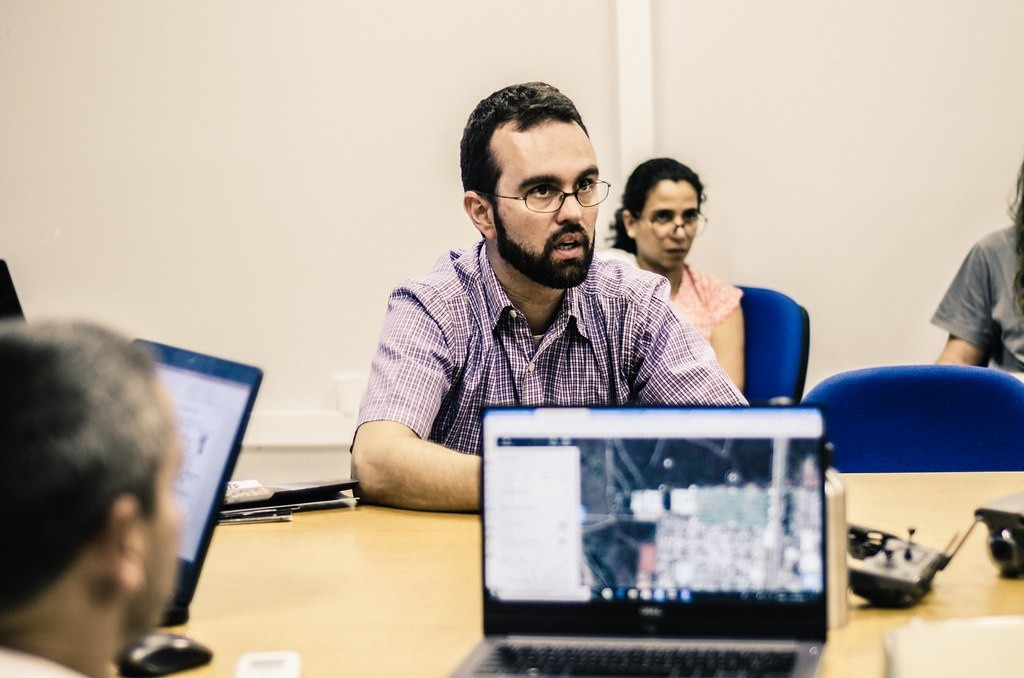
[476,178,611,214]
[633,211,707,241]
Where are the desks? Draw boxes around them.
[108,467,1024,678]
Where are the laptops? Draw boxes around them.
[443,404,827,678]
[220,478,362,511]
[132,337,264,627]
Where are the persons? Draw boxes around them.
[350,80,753,510]
[0,318,185,678]
[602,156,747,394]
[930,159,1024,378]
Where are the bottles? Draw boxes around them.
[820,443,851,631]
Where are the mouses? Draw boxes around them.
[119,632,213,678]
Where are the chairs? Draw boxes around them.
[790,367,1023,472]
[737,287,809,405]
